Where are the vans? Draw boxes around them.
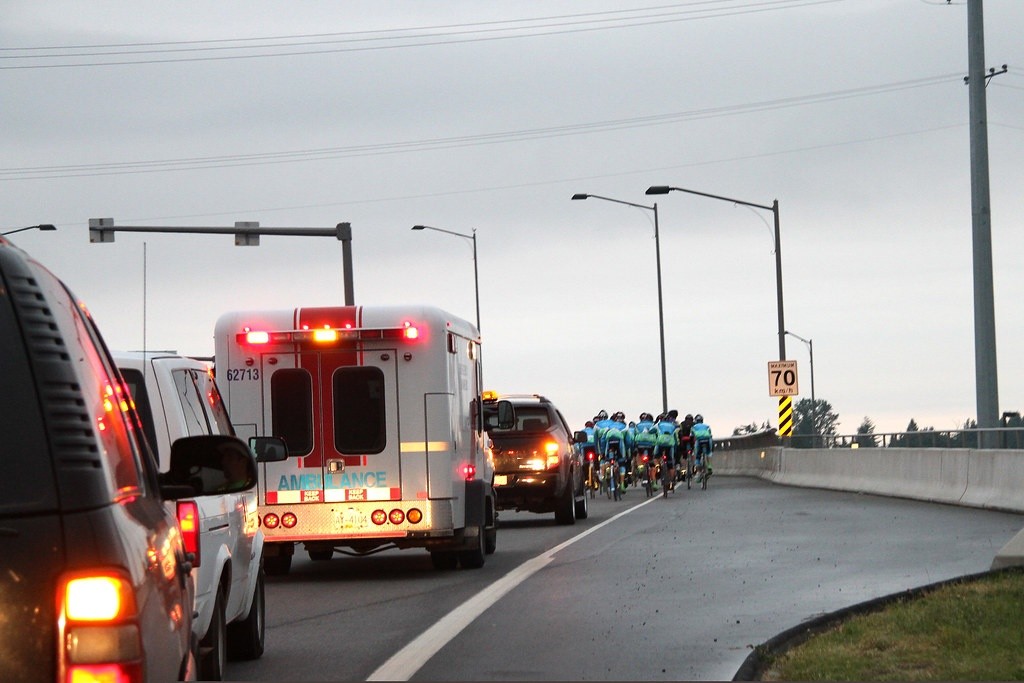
[109,348,290,683]
[0,246,259,683]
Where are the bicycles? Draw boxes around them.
[577,444,711,504]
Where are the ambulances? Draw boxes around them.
[214,306,498,581]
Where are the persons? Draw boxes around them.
[575,410,714,494]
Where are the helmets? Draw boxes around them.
[583,409,710,427]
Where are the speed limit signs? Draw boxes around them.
[767,360,798,396]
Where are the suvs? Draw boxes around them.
[480,392,589,527]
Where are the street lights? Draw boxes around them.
[572,193,667,414]
[777,331,817,447]
[644,185,794,437]
[413,225,480,334]
